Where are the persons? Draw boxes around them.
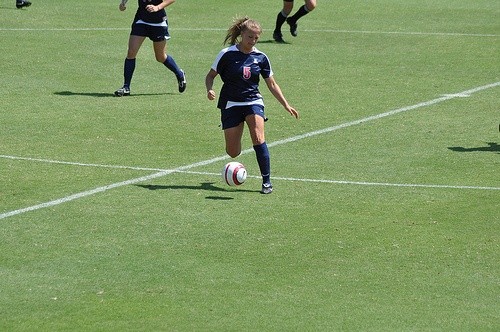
[206,17,300,194]
[273,0,317,43]
[115,0,186,96]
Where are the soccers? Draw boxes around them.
[221,162,246,186]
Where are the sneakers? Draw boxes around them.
[273,28,284,44]
[114,85,130,97]
[15,0,31,9]
[286,17,298,37]
[261,181,274,194]
[176,69,187,93]
